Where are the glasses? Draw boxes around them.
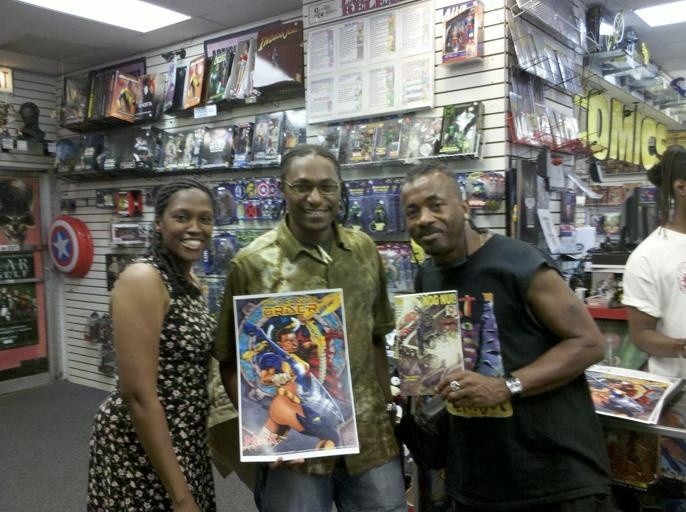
[282,180,343,195]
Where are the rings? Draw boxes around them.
[449,379,461,392]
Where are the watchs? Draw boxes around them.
[504,372,524,402]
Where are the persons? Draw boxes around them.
[392,164,608,510]
[20,103,45,141]
[214,146,408,511]
[620,144,686,380]
[88,179,218,512]
